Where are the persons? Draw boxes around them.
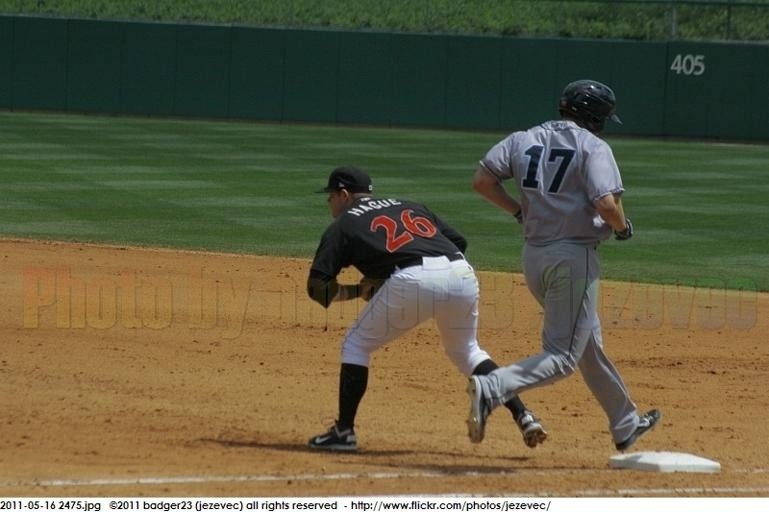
[462,77,659,449]
[307,166,547,453]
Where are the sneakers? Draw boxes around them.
[308,423,357,453]
[464,375,490,444]
[615,407,661,451]
[514,411,546,449]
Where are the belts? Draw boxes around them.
[389,253,463,276]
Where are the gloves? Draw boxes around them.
[614,217,633,241]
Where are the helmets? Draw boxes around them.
[558,78,623,134]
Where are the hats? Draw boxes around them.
[314,165,374,195]
